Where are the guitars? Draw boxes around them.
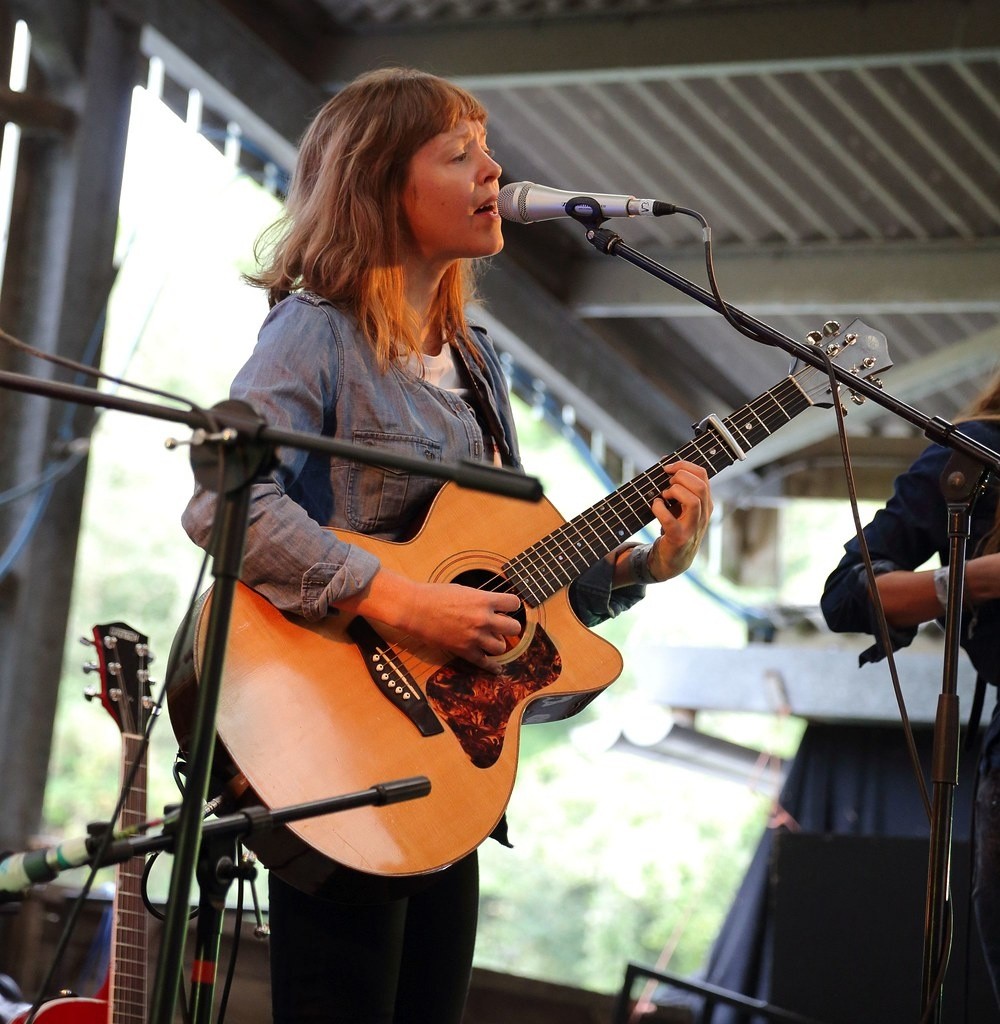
[164,315,897,905]
[11,620,158,1023]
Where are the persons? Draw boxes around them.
[821,370,1000,1002]
[184,71,714,1024]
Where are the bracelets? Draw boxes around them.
[933,565,947,610]
[631,544,659,585]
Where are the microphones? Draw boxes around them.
[0,833,115,892]
[497,181,677,224]
